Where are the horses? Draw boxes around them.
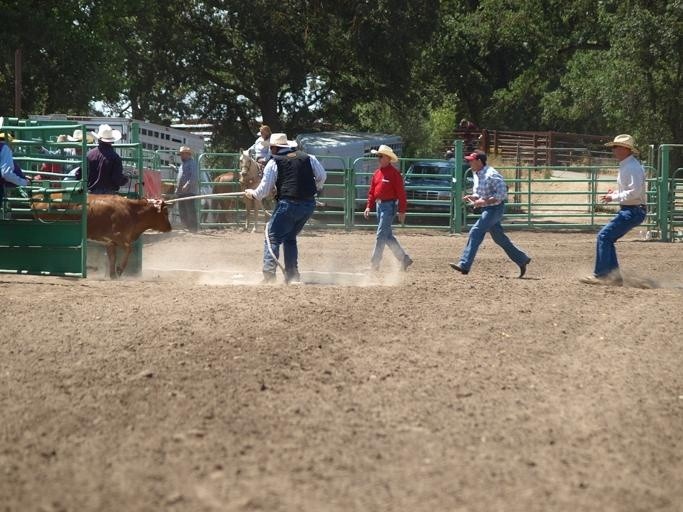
[200,147,278,234]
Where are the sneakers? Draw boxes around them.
[399,255,413,271]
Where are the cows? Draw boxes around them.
[29,191,174,281]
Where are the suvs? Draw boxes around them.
[402,160,473,215]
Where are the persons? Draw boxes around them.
[453,116,478,148]
[172,145,200,234]
[238,123,272,167]
[53,132,67,142]
[243,131,327,282]
[73,122,130,194]
[0,116,31,188]
[447,148,532,280]
[576,133,648,287]
[361,144,414,275]
[34,128,94,175]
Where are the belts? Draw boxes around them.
[278,196,314,201]
[620,204,647,209]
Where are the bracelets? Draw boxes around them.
[251,192,256,199]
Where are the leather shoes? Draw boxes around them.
[449,263,469,274]
[519,258,530,277]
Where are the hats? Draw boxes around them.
[370,145,399,164]
[91,124,122,143]
[464,150,487,162]
[261,133,298,148]
[176,146,194,156]
[603,134,640,156]
[66,129,94,143]
